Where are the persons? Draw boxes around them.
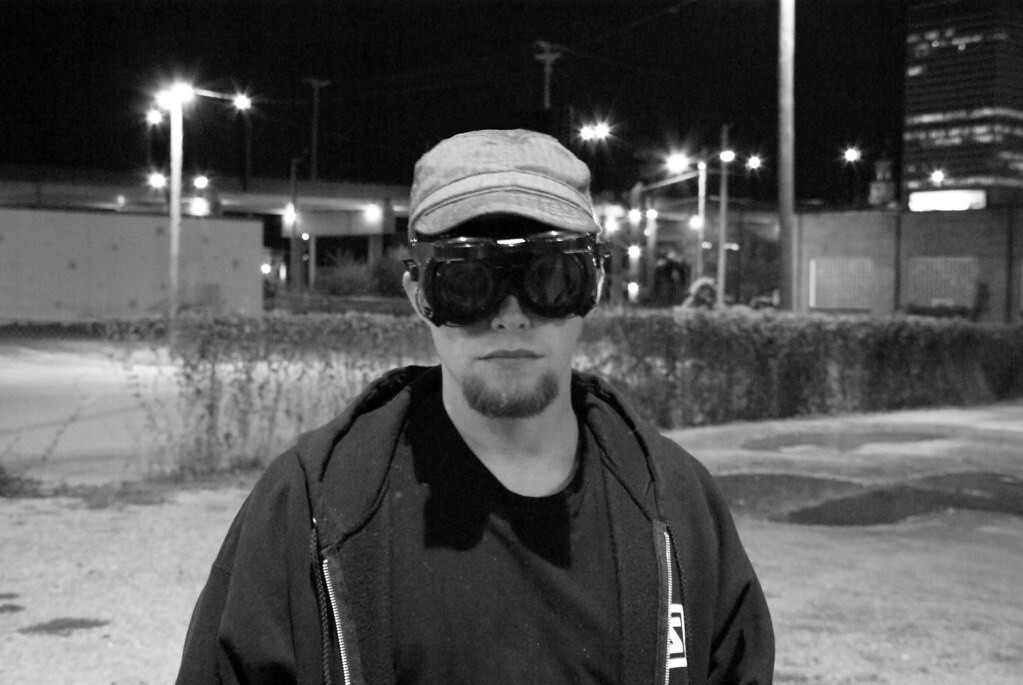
[174,128,775,685]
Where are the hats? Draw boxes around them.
[409,129,602,237]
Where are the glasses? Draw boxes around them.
[406,235,600,327]
